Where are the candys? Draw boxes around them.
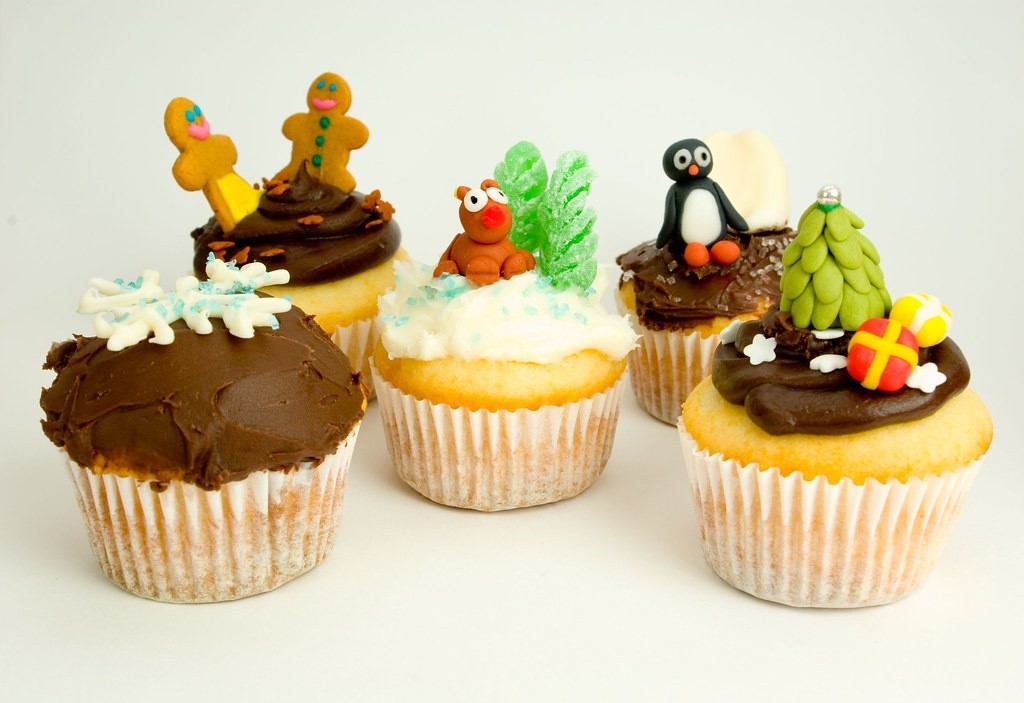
[540,133,598,292]
[495,141,549,255]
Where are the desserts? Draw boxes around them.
[40,72,994,609]
